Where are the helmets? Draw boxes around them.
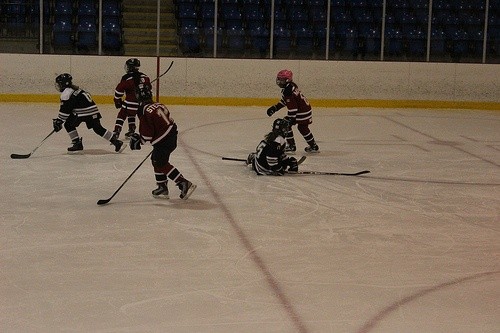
[136,84,152,102]
[273,119,290,139]
[276,70,292,88]
[124,58,140,73]
[54,73,73,92]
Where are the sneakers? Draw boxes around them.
[180,180,197,201]
[287,161,298,176]
[67,137,87,154]
[110,131,135,145]
[246,152,253,165]
[303,141,320,154]
[282,143,296,155]
[152,187,171,200]
[110,134,128,154]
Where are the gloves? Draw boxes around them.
[52,118,64,132]
[267,106,276,116]
[288,157,297,166]
[282,119,288,128]
[115,100,123,108]
[276,163,285,175]
[130,133,141,150]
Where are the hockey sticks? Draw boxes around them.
[150,60,174,84]
[95,150,153,205]
[281,170,370,176]
[222,156,307,166]
[9,130,55,160]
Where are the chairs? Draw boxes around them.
[173,0,500,65]
[0,0,125,56]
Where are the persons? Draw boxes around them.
[110,57,153,145]
[127,84,197,200]
[246,119,299,175]
[53,73,127,154]
[266,70,320,153]
